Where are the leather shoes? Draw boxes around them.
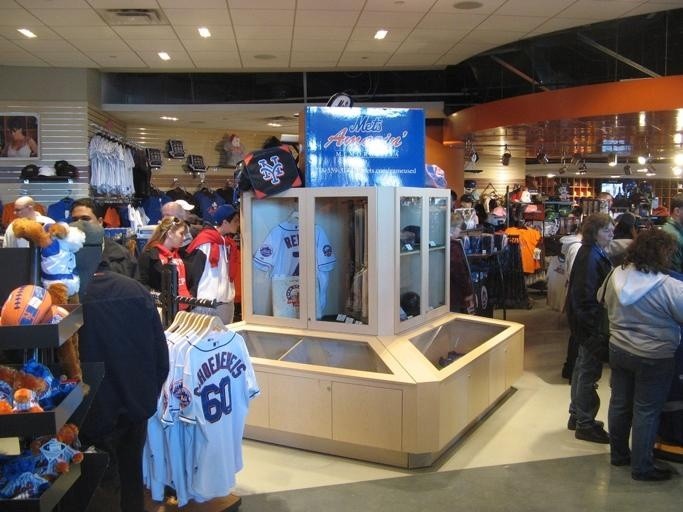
[567,420,672,481]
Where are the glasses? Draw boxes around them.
[71,216,92,223]
[9,128,16,133]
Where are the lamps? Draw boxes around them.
[500,141,656,179]
[464,141,479,165]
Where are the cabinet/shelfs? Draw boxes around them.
[269,374,403,452]
[245,372,268,429]
[439,356,489,448]
[243,187,377,335]
[526,176,682,216]
[394,187,451,335]
[489,333,522,404]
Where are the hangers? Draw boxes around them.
[96,125,147,151]
[165,295,230,348]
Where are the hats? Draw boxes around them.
[174,199,194,211]
[461,195,475,203]
[21,160,77,176]
[622,212,636,226]
[212,204,238,225]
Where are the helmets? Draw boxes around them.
[402,224,419,243]
[401,292,419,315]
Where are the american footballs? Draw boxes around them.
[2,285,69,326]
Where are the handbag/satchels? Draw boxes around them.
[583,328,608,362]
[238,143,302,200]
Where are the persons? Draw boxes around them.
[486,198,506,225]
[561,193,683,481]
[451,191,457,209]
[458,195,480,231]
[1,115,38,159]
